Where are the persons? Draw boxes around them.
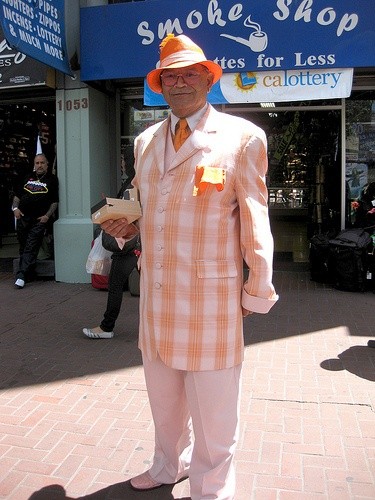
[100,35,279,500]
[11,155,58,288]
[82,148,138,338]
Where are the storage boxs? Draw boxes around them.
[91,189,142,225]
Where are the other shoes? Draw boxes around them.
[129,470,163,490]
[82,327,114,339]
[14,278,26,288]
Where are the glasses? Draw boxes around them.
[160,68,202,86]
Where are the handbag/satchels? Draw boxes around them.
[84,230,111,277]
[102,227,139,252]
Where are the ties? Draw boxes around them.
[172,119,192,152]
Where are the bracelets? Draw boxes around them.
[12,207,18,211]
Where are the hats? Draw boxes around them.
[145,33,223,94]
